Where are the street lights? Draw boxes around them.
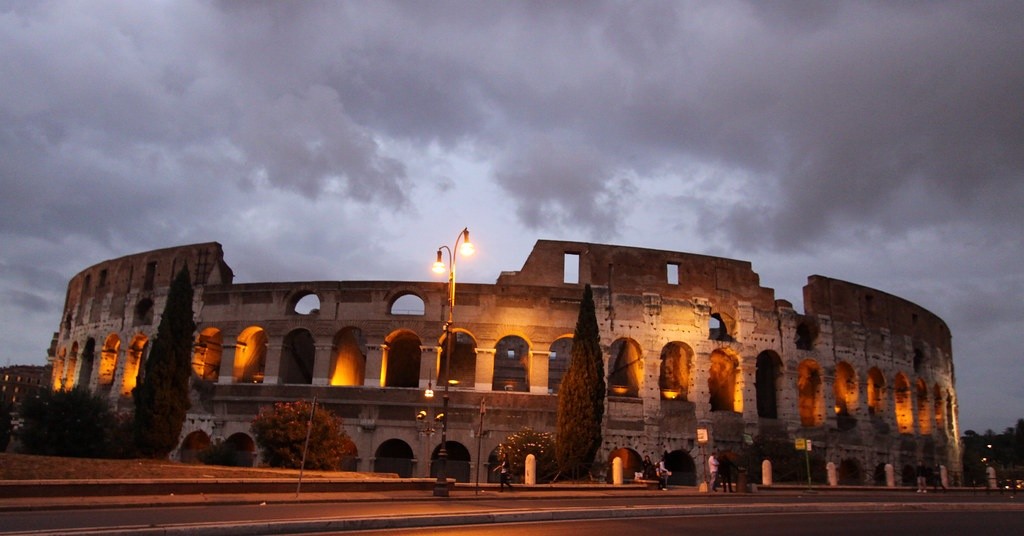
[432,226,475,497]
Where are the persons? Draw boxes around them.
[493,453,513,493]
[708,452,739,493]
[641,455,669,490]
[916,460,927,494]
[931,463,947,494]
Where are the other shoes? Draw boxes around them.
[923,490,927,493]
[662,488,668,490]
[917,490,921,492]
